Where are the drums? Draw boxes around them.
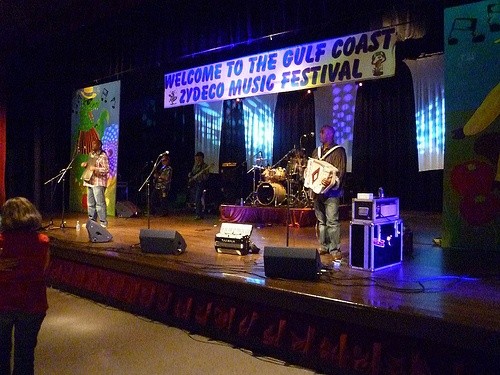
[256,181,286,205]
[265,158,308,182]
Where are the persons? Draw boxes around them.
[80,139,109,228]
[188,151,209,223]
[149,155,173,218]
[293,151,309,191]
[304,125,347,260]
[0,197,50,375]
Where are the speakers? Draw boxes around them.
[85,219,113,243]
[139,229,188,256]
[263,246,322,281]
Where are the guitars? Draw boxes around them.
[150,165,166,194]
[187,161,215,188]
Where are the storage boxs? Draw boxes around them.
[351,196,400,224]
[348,218,404,272]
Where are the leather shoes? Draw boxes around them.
[331,251,341,261]
[320,248,330,255]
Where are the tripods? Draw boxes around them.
[43,149,81,232]
[278,181,310,208]
[243,168,257,206]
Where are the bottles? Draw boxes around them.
[378,187,384,198]
[76,221,80,231]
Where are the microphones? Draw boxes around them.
[160,151,169,156]
[61,166,73,171]
[261,152,263,159]
[303,132,314,137]
[78,146,87,149]
